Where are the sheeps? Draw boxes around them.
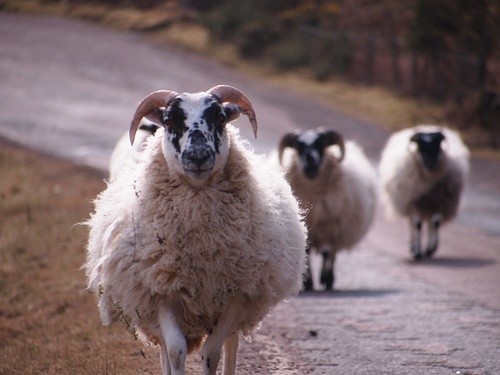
[69,84,313,374]
[378,123,470,260]
[270,126,380,290]
